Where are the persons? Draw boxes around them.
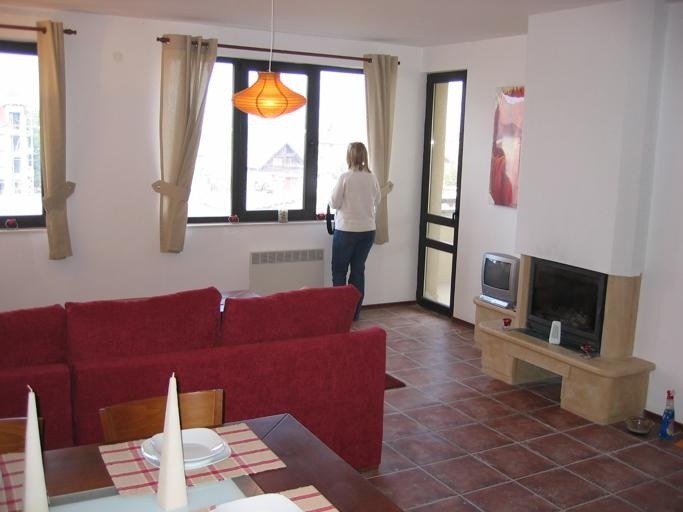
[327,141,381,321]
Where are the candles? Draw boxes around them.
[157,373,188,510]
[24,385,48,512]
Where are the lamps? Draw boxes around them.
[232,0,306,118]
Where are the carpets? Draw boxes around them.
[385,373,406,392]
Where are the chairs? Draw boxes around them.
[1,389,223,453]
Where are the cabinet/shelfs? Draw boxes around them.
[473,296,656,427]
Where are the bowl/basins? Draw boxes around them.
[623,414,655,434]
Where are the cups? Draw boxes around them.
[277,208,288,223]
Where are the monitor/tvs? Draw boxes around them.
[526,257,607,354]
[481,252,520,309]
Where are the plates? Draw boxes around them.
[209,491,302,512]
[140,425,234,471]
